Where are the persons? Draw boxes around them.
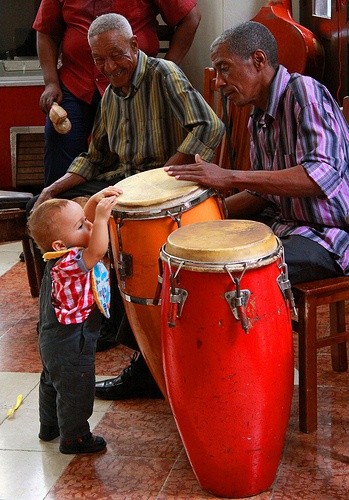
[26,185,124,455]
[93,20,348,399]
[30,12,228,350]
[32,0,201,186]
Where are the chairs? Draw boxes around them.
[204,66,255,198]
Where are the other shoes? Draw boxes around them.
[94,373,163,400]
[39,430,60,441]
[58,435,106,454]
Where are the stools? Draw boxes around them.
[287,276,349,435]
[0,190,44,297]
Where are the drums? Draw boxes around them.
[160,219,295,499]
[108,166,226,406]
